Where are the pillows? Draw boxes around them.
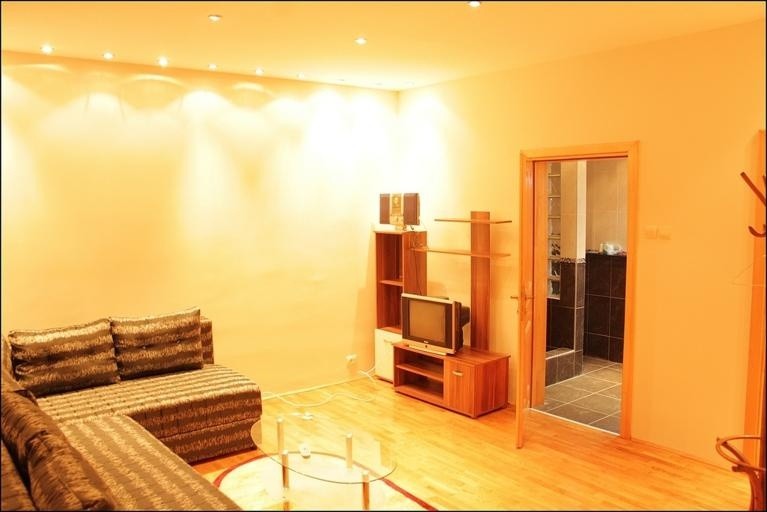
[2,304,207,397]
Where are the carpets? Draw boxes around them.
[210,452,437,512]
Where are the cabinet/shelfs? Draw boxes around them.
[373,212,512,418]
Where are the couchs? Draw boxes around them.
[0,312,264,510]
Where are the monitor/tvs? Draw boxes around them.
[401,292,470,356]
